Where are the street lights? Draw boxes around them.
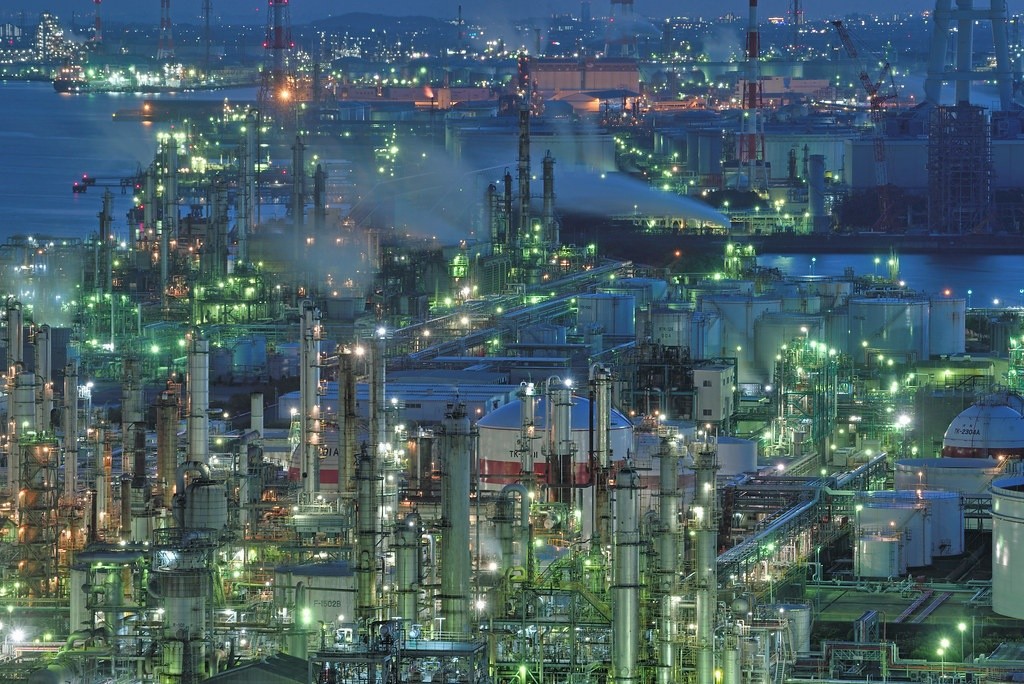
[941,638,950,662]
[865,449,871,491]
[937,648,944,676]
[967,289,972,307]
[800,326,810,338]
[856,503,862,581]
[812,257,816,276]
[958,621,966,663]
[918,471,922,484]
[874,257,879,273]
